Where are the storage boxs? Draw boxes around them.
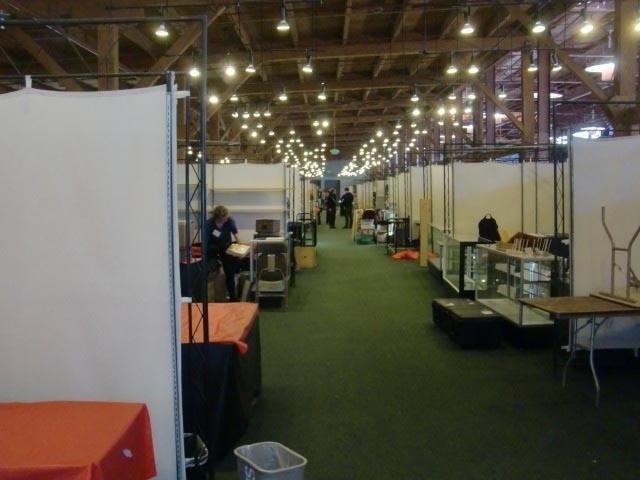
[294,247,316,268]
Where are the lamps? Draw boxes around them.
[410,86,420,117]
[446,49,479,100]
[154,3,169,38]
[276,0,291,31]
[244,49,327,101]
[459,1,475,34]
[530,1,593,35]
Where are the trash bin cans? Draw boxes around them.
[233,441,308,480]
[183,433,209,480]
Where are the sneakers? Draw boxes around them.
[330,226,352,228]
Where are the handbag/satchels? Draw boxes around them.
[479,214,501,241]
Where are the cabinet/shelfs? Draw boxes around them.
[443,233,559,298]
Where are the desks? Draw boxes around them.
[522,206,640,404]
[181,302,261,462]
[2,402,146,478]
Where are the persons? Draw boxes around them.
[316,187,356,231]
[202,205,239,300]
